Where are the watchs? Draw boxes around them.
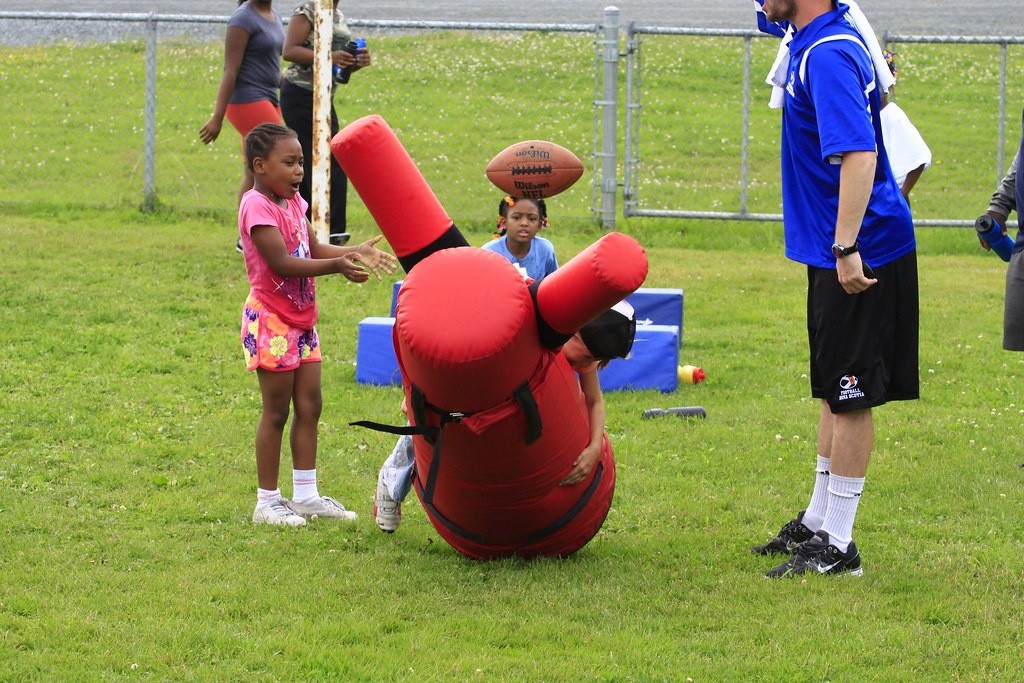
[831,242,860,258]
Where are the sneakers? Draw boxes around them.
[251,496,355,527]
[372,467,403,534]
[751,511,862,583]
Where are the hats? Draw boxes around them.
[581,298,638,360]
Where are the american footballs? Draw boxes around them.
[486,140,585,199]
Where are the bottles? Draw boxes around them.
[676,363,707,386]
[641,406,707,421]
[333,36,367,73]
[336,40,358,84]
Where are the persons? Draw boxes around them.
[474,192,561,286]
[199,1,286,255]
[374,299,637,534]
[282,1,371,235]
[236,122,397,529]
[876,55,932,208]
[761,0,921,587]
[977,103,1024,353]
